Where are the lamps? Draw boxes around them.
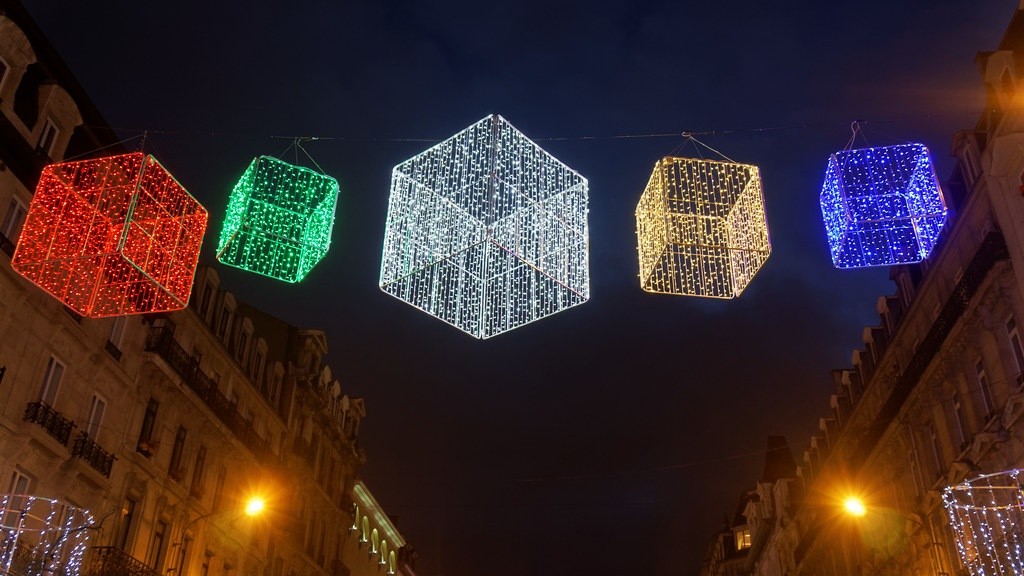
[167,487,272,573]
[834,489,943,549]
